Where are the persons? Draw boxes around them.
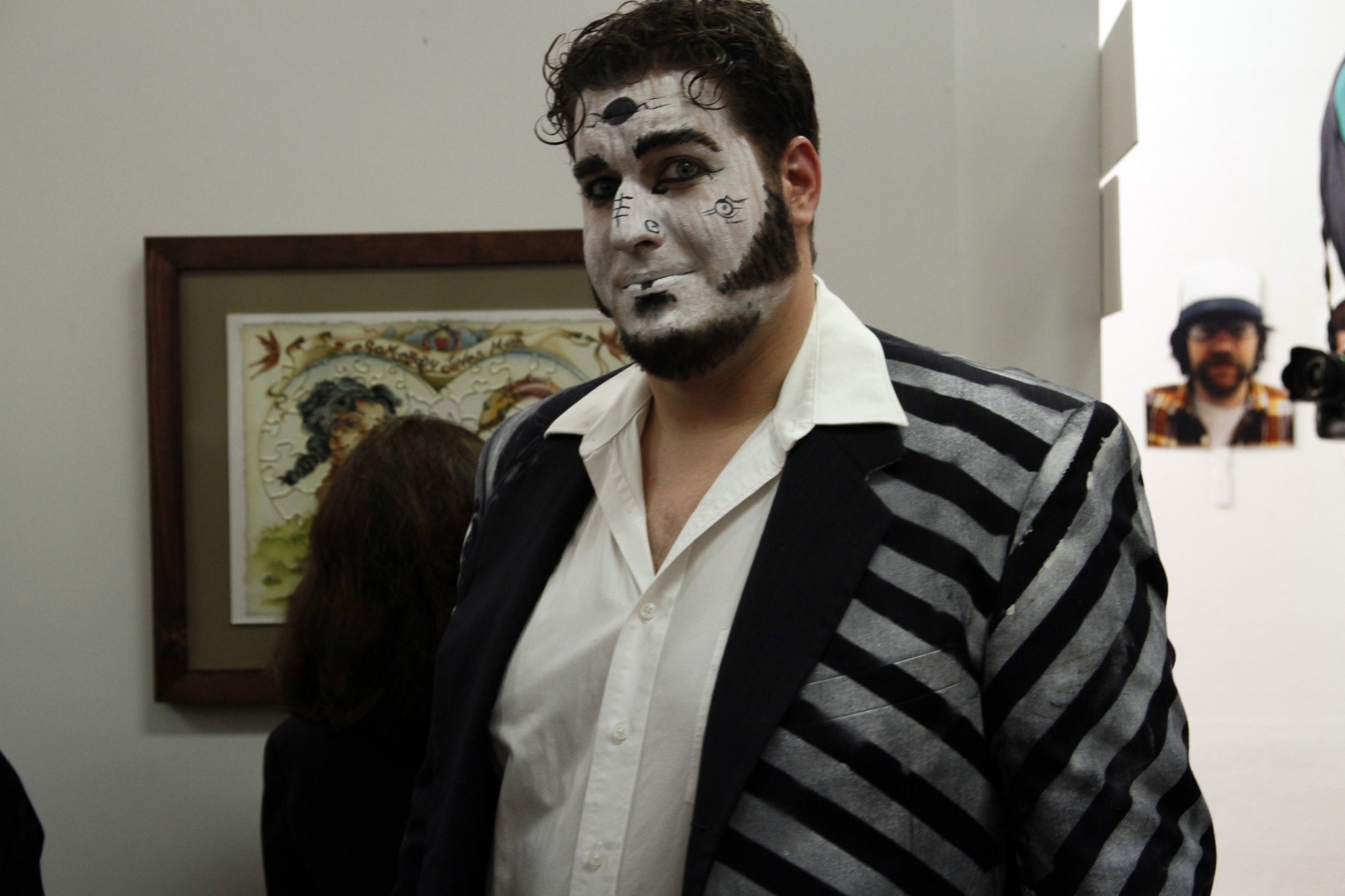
[1320,55,1345,357]
[262,415,484,896]
[1148,269,1295,449]
[392,0,1216,896]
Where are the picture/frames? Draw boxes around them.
[144,228,635,707]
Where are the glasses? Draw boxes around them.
[1185,319,1251,342]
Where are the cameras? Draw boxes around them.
[1283,345,1345,415]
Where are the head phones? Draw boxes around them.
[1165,298,1266,371]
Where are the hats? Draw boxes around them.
[1177,297,1271,328]
